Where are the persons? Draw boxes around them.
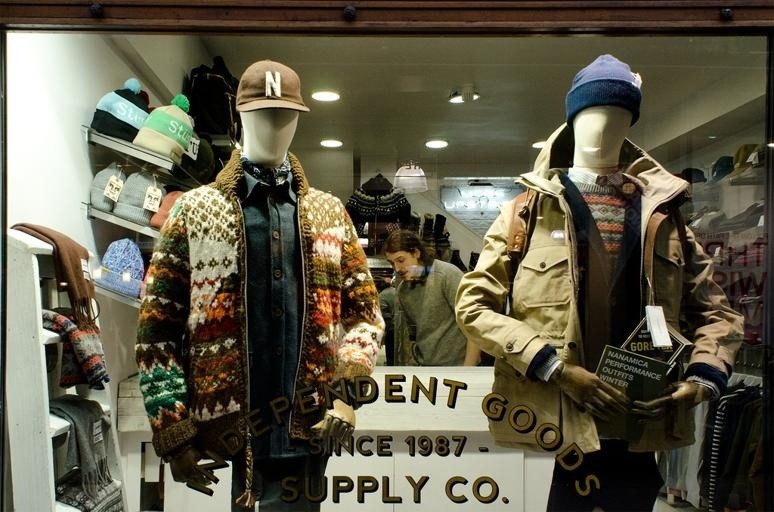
[375,274,395,364]
[135,60,386,512]
[453,53,745,511]
[385,230,482,366]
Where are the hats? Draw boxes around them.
[674,144,765,184]
[94,238,145,298]
[566,54,641,128]
[236,59,311,112]
[91,78,215,229]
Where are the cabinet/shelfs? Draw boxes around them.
[5,229,130,512]
[80,123,206,308]
[677,165,767,350]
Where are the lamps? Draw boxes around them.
[393,162,429,195]
